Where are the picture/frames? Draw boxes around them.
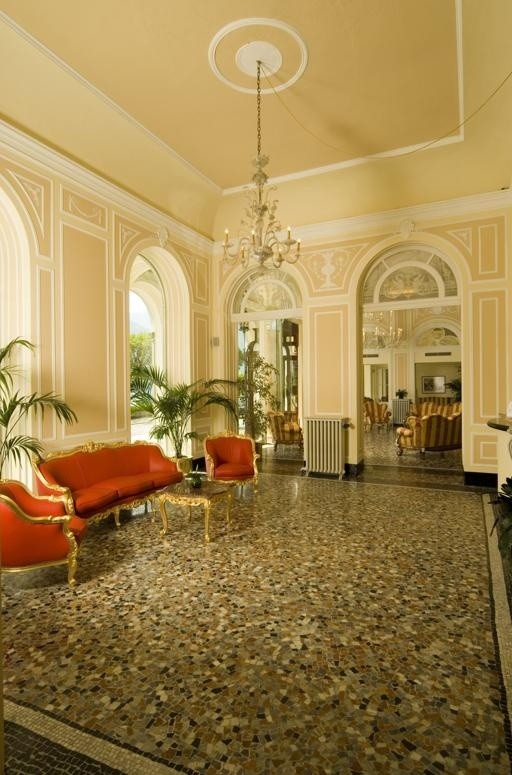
[421,375,446,394]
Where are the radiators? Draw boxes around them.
[302,418,345,480]
[391,400,409,427]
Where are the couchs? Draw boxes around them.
[31,438,184,528]
[394,402,462,460]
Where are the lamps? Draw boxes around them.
[219,59,302,270]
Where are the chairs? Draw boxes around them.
[0,478,87,587]
[266,410,303,451]
[202,428,261,496]
[362,397,392,433]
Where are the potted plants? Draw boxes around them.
[395,389,408,399]
[237,345,281,462]
[128,357,244,475]
